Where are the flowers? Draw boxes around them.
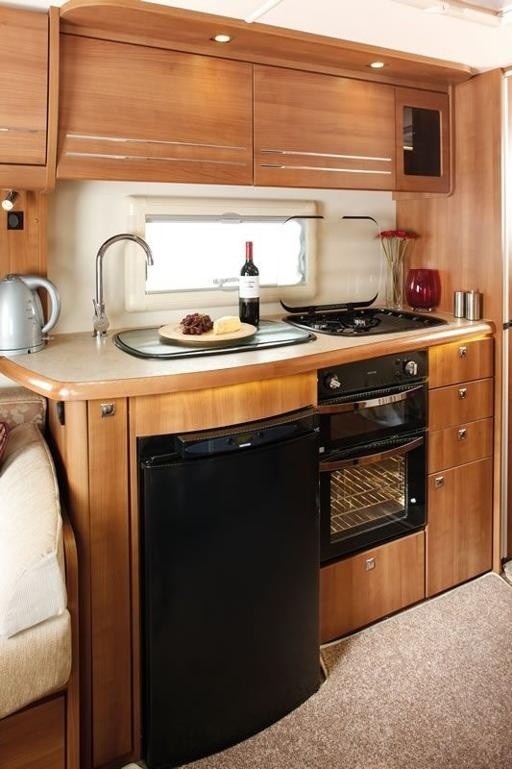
[373,230,420,303]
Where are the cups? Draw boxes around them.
[454,291,464,318]
[465,290,481,321]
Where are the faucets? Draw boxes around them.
[93,232,154,341]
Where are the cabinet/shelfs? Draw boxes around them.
[1,2,56,191]
[56,26,395,192]
[427,333,494,600]
[396,86,453,193]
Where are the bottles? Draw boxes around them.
[239,240,260,328]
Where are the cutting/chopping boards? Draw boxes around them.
[118,319,310,356]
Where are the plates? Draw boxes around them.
[157,319,258,344]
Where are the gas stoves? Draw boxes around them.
[282,307,448,336]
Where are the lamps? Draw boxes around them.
[2,190,21,211]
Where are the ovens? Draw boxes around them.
[317,347,430,569]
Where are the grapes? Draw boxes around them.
[179,313,213,334]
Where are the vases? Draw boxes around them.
[406,268,441,313]
[385,261,404,311]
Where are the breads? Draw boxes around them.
[214,316,241,335]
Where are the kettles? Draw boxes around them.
[0,273,61,358]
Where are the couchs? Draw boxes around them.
[0,386,79,766]
